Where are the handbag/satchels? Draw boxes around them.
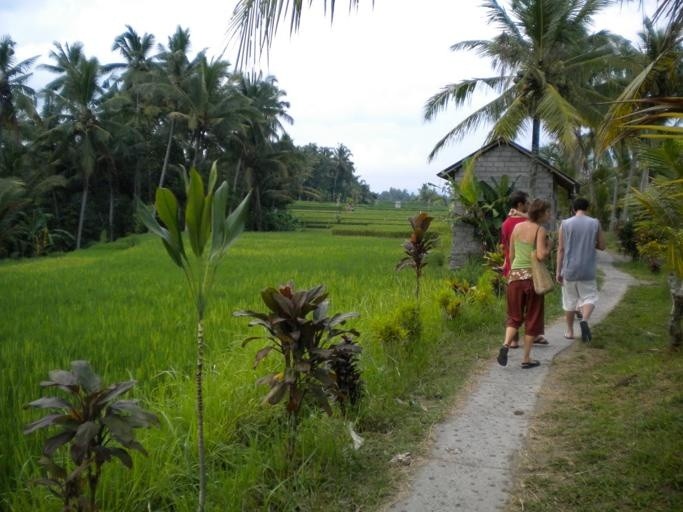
[532,250,555,295]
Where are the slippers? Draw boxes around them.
[580,321,592,342]
[564,333,573,339]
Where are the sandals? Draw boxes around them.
[497,344,509,365]
[533,336,548,344]
[521,360,540,369]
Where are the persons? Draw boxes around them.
[491,208,529,273]
[556,197,606,341]
[500,191,550,349]
[496,197,556,370]
